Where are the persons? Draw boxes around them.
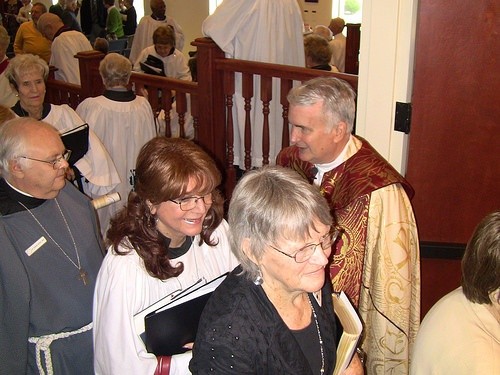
[37,13,92,86]
[127,0,185,71]
[150,0,306,170]
[93,137,241,375]
[409,212,500,375]
[6,52,120,202]
[276,77,420,375]
[93,37,108,53]
[187,164,364,375]
[132,23,192,105]
[0,118,106,375]
[78,52,156,221]
[303,18,347,73]
[0,0,137,112]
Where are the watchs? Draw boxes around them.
[357,347,366,364]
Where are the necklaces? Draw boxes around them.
[19,197,90,287]
[24,106,43,122]
[306,295,325,375]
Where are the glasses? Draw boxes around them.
[263,226,340,264]
[15,148,72,170]
[168,190,219,211]
[29,11,43,15]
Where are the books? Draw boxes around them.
[140,54,166,90]
